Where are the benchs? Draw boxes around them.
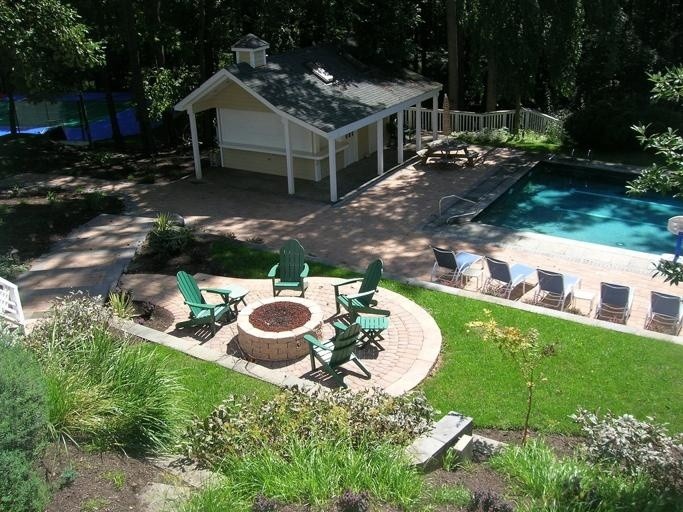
[420,148,429,166]
[466,150,479,167]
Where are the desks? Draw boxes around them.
[424,140,474,167]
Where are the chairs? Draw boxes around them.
[176,239,391,388]
[429,246,683,338]
